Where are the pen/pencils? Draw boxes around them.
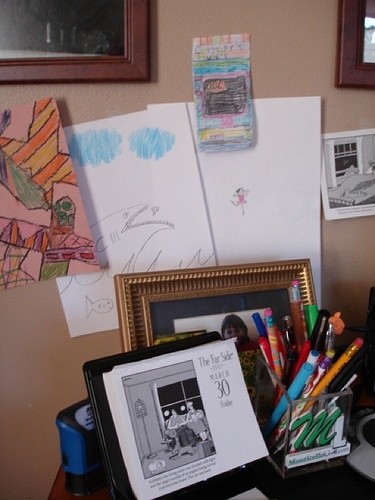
[253,281,364,453]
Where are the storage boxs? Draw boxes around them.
[253,352,354,478]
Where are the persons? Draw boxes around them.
[220,313,251,346]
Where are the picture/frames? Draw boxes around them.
[114,258,317,430]
[82,331,268,500]
[335,0,375,89]
[0,0,150,83]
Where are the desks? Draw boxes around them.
[47,384,375,500]
